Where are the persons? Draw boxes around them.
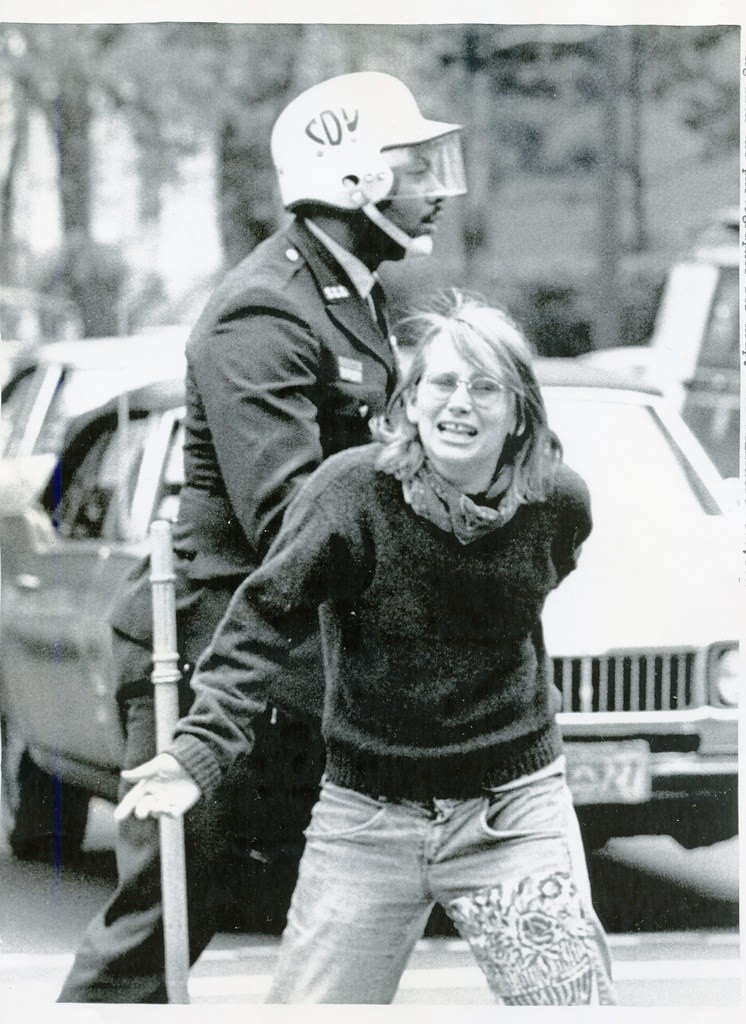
[57,71,470,1004]
[115,287,618,1006]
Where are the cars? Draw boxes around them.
[0,202,740,861]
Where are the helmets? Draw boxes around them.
[270,70,460,210]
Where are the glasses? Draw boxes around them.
[423,372,506,408]
